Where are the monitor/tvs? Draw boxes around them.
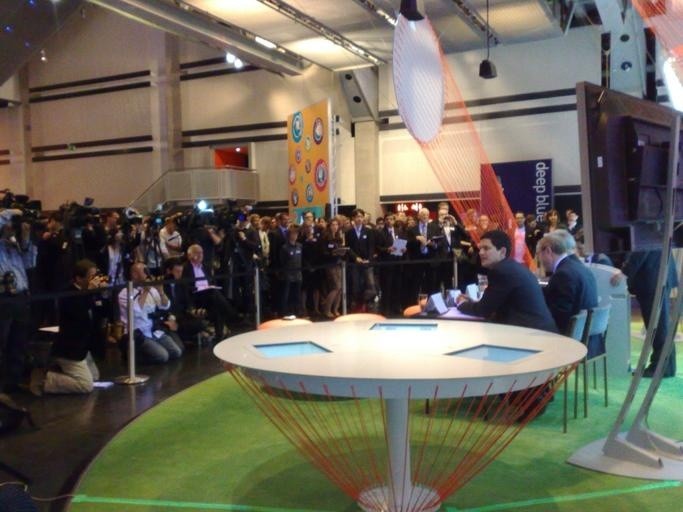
[575,81,682,255]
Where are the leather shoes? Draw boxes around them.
[632,364,673,377]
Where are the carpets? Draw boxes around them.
[68,320,682,511]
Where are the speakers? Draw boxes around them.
[336,64,379,118]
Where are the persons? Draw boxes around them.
[2,204,184,420]
[625,229,679,381]
[164,204,469,341]
[456,208,611,421]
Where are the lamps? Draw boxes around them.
[480,1,497,79]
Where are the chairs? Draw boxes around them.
[581,305,612,418]
[559,309,587,433]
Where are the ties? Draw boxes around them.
[422,224,427,253]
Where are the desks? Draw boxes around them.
[212,320,589,512]
[403,293,485,320]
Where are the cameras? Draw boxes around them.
[94,270,109,283]
[147,273,175,283]
[444,215,451,219]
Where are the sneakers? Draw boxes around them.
[30,367,46,398]
